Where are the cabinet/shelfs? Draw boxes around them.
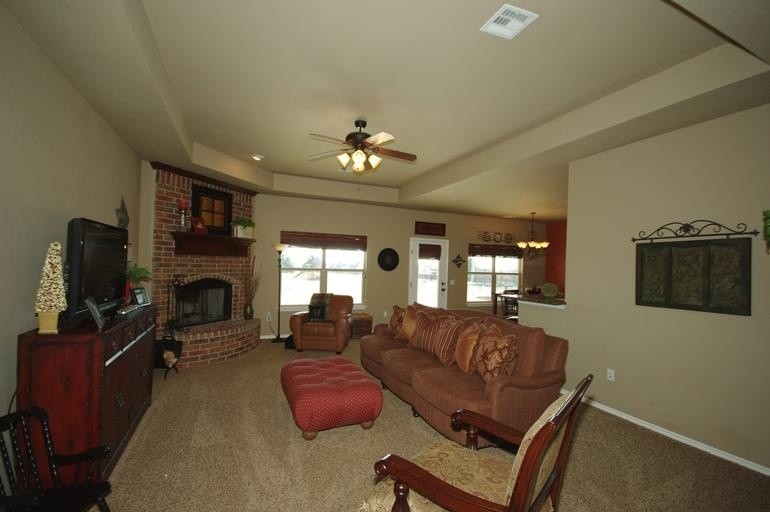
[16,306,155,495]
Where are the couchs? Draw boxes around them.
[360,302,569,449]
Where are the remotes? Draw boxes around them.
[117,304,139,315]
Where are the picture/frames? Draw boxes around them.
[131,286,151,305]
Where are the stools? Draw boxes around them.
[280,356,384,439]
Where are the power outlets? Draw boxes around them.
[267,313,271,321]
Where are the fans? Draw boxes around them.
[308,120,417,162]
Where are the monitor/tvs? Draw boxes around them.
[59,217,128,332]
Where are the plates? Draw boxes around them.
[483,232,490,241]
[542,282,558,297]
[504,233,514,245]
[494,232,502,242]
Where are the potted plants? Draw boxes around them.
[125,262,152,303]
[231,216,255,238]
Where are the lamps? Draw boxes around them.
[517,212,550,259]
[272,241,285,342]
[337,148,382,172]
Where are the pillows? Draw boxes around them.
[388,302,546,385]
[308,294,333,321]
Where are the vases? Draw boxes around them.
[244,304,254,319]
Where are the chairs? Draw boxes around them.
[154,337,183,379]
[0,408,112,512]
[492,290,522,321]
[290,293,353,355]
[355,372,593,512]
